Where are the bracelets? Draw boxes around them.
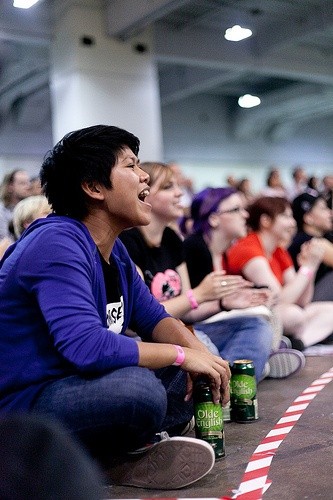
[186,288,199,309]
[218,298,233,312]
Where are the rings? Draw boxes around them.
[221,281,227,287]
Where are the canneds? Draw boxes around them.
[192,376,226,461]
[230,359,259,423]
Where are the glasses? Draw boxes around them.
[213,204,248,215]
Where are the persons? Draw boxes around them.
[0,159,333,416]
[171,345,185,366]
[0,125,240,490]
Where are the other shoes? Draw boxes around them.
[259,362,269,382]
[268,348,304,379]
[103,431,215,490]
[280,336,292,350]
[180,416,196,436]
